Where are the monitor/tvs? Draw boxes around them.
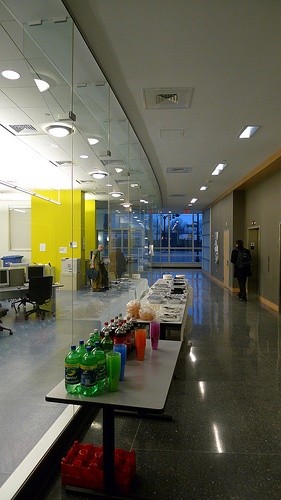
[0,269,10,288]
[25,265,45,282]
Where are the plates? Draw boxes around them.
[159,306,183,322]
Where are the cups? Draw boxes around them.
[105,352,121,391]
[135,329,146,361]
[150,322,160,350]
[113,344,127,382]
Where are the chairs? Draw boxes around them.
[24,276,55,321]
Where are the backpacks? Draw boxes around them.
[236,248,250,268]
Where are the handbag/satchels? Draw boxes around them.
[88,268,98,280]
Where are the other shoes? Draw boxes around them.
[93,288,100,292]
[239,296,247,302]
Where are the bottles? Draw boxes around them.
[65,345,81,394]
[92,341,106,394]
[75,341,88,355]
[85,314,137,359]
[80,346,99,396]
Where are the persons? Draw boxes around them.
[231,240,253,302]
[90,244,105,292]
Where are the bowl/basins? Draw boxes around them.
[146,279,187,304]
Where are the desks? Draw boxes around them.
[45,276,190,500]
[0,279,64,322]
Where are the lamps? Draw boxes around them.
[120,203,133,208]
[110,191,124,198]
[44,125,75,137]
[89,170,109,180]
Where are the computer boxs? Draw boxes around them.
[9,269,24,287]
